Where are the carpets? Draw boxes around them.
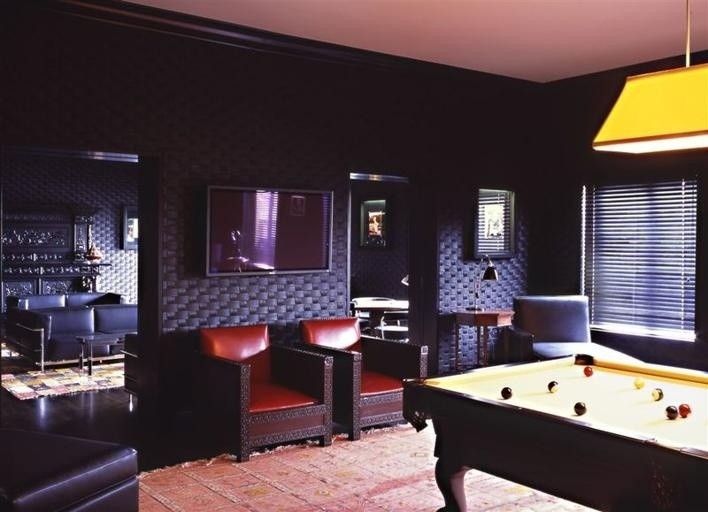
[0,339,125,401]
[138,419,608,512]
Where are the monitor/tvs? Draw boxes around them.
[201,184,335,278]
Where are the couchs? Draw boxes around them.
[0,293,138,373]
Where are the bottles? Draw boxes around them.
[4,283,32,297]
[44,280,72,295]
[77,227,87,251]
[84,243,102,261]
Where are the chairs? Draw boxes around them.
[350,297,410,343]
[0,427,139,512]
[502,294,643,362]
[294,319,428,440]
[191,324,334,462]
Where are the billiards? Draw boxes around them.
[574,402,586,415]
[651,388,664,401]
[501,387,512,399]
[634,377,645,389]
[548,381,559,392]
[584,367,592,376]
[666,404,691,420]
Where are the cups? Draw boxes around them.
[3,228,53,245]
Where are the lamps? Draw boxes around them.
[466,253,499,311]
[592,0,708,155]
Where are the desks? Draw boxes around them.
[402,353,708,512]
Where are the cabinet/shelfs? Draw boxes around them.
[0,201,112,315]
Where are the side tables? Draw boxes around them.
[452,307,516,371]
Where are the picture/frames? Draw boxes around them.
[358,196,393,251]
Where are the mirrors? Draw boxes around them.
[464,183,521,260]
